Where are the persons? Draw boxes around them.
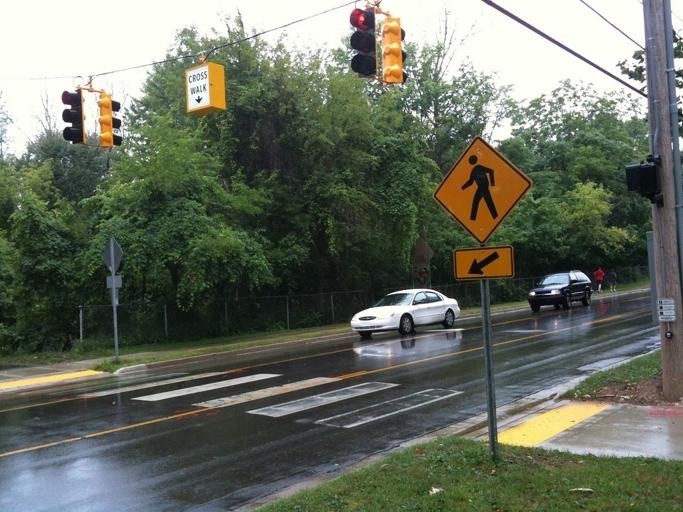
[459,153,501,223]
[593,266,605,294]
[607,268,619,293]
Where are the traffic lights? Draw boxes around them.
[61,87,85,144]
[381,18,407,85]
[98,92,122,148]
[621,150,663,205]
[348,7,374,80]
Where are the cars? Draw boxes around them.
[351,289,462,337]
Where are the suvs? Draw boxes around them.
[527,270,593,312]
